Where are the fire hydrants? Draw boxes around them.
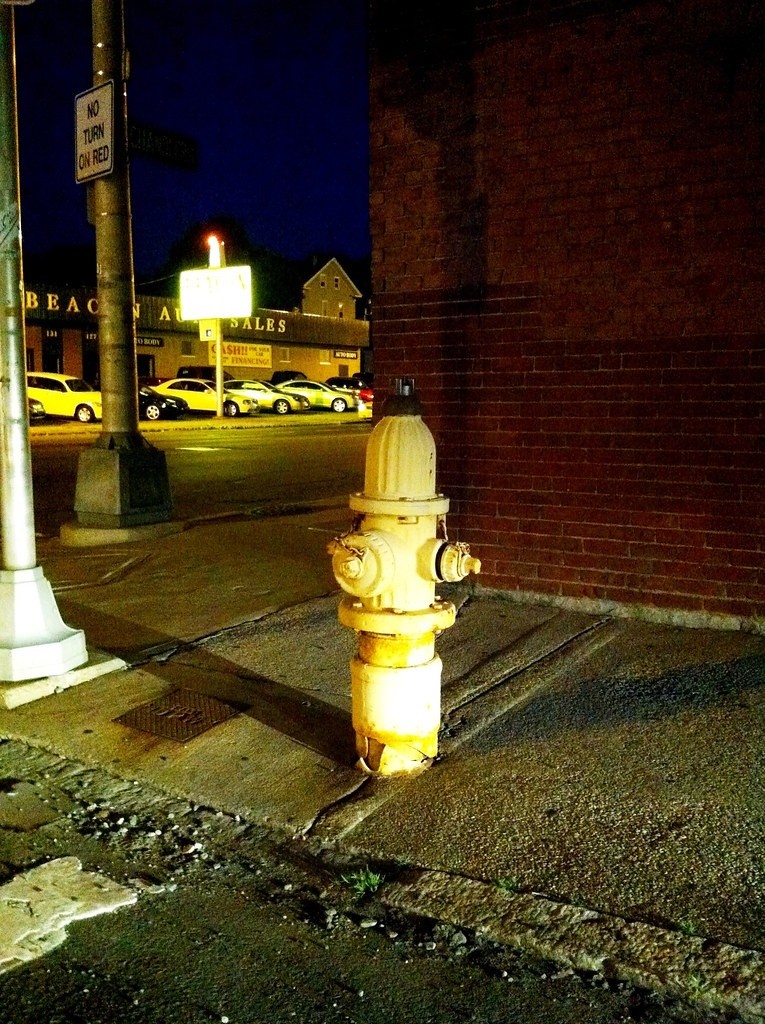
[325,380,483,780]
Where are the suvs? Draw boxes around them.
[270,371,308,384]
[326,376,373,402]
[176,366,235,383]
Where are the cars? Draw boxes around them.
[277,379,358,413]
[134,383,190,419]
[224,379,312,414]
[26,372,103,423]
[148,376,261,417]
